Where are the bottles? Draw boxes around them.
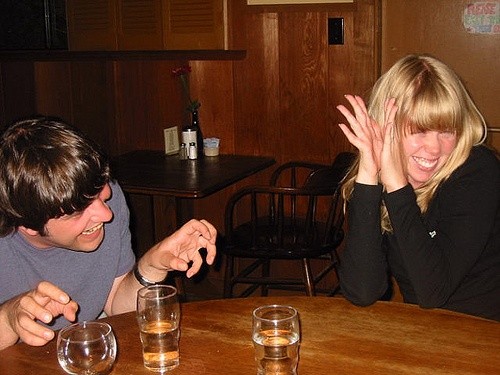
[178,142,187,160]
[189,142,197,159]
[181,129,197,157]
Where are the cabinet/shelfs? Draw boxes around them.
[164,0,247,62]
[115,0,162,59]
[63,0,117,61]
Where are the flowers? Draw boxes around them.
[171,61,202,112]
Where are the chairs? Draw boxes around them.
[222,149,352,298]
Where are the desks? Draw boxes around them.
[104,148,278,302]
[0,295,500,375]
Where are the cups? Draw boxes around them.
[135,285,180,372]
[250,304,301,375]
[204,147,220,156]
[56,321,118,375]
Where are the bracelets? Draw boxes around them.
[133,262,165,289]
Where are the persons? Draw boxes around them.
[336,55,500,322]
[0,118,218,350]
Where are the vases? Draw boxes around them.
[189,110,204,161]
